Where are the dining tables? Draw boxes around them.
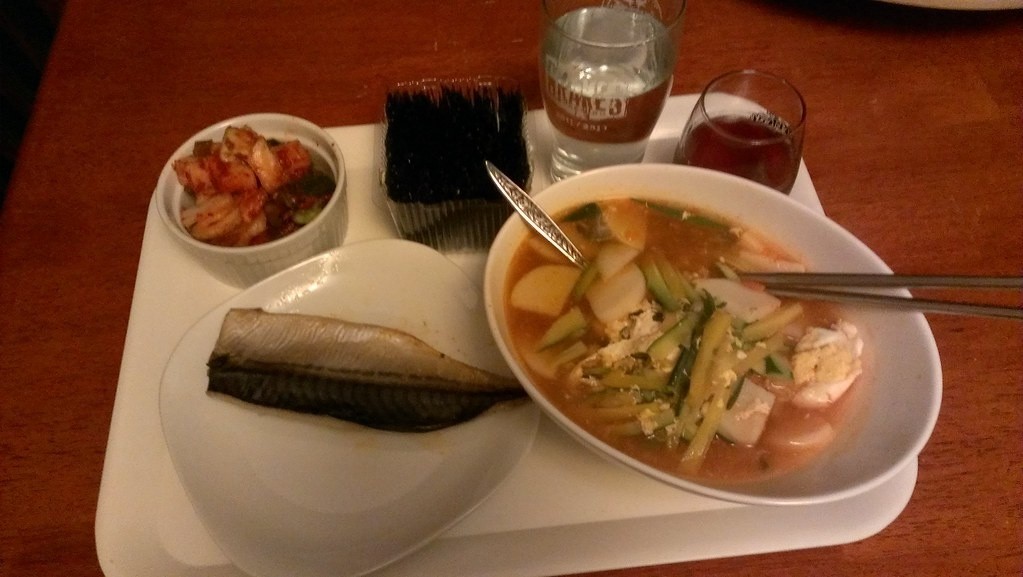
[0,1,1023,576]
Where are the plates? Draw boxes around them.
[158,237,540,577]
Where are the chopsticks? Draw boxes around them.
[742,274,1023,323]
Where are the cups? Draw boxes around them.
[673,69,806,197]
[539,0,688,185]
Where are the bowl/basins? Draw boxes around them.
[483,164,945,506]
[156,111,349,289]
[382,76,536,255]
